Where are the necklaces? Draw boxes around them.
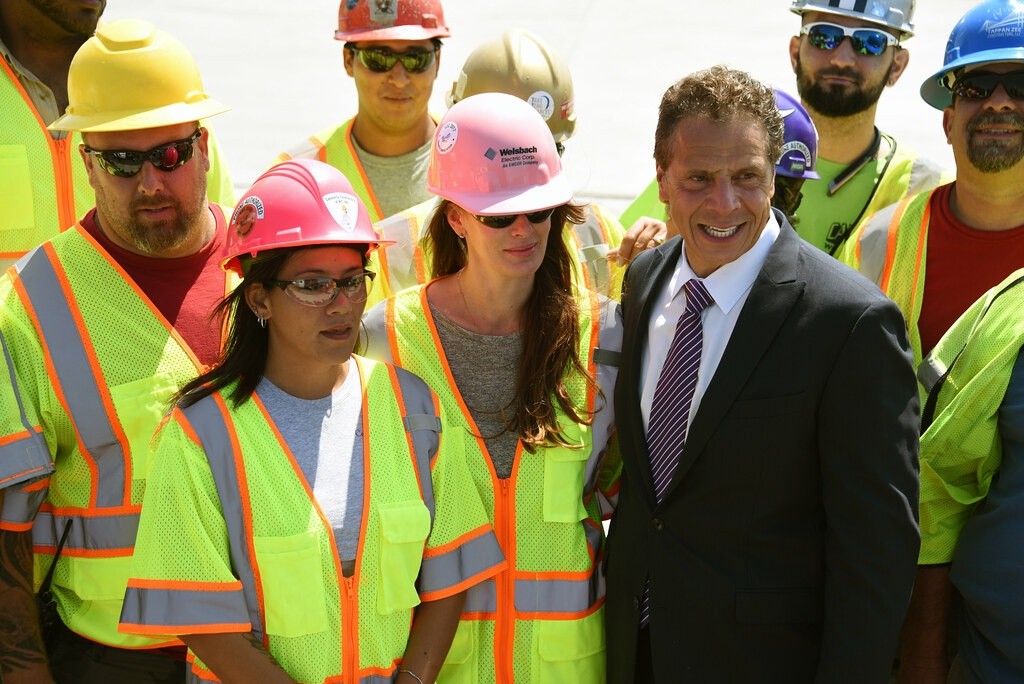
[458,266,470,312]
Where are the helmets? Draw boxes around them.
[41,21,229,132]
[764,87,822,183]
[918,0,1024,110]
[789,0,916,42]
[425,92,576,216]
[445,34,579,148]
[219,157,399,275]
[332,0,452,42]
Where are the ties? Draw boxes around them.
[637,279,716,629]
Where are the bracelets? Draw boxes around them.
[399,669,423,684]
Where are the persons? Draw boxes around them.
[895,347,1024,684]
[358,93,626,684]
[837,0,1024,684]
[620,0,950,255]
[0,0,239,275]
[362,32,666,310]
[1,18,243,684]
[606,64,924,683]
[118,160,509,684]
[262,0,449,218]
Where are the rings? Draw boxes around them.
[652,238,662,245]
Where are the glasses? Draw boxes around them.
[798,22,902,55]
[949,69,1024,102]
[259,271,378,307]
[346,45,442,74]
[83,128,203,178]
[469,208,555,228]
